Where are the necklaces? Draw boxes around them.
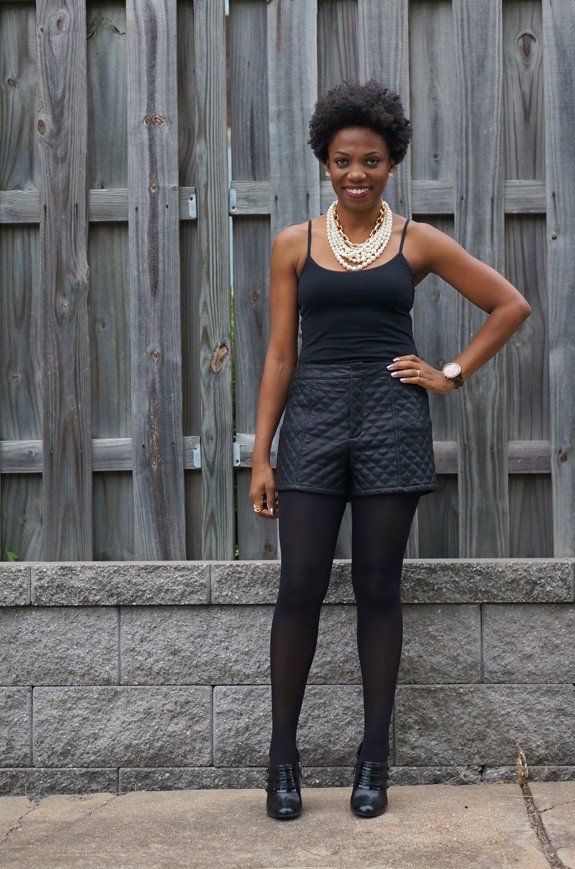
[327,199,393,271]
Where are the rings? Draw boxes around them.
[416,370,420,377]
[253,503,263,513]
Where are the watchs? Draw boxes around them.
[442,362,464,389]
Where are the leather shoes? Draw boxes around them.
[266,748,303,819]
[349,741,390,818]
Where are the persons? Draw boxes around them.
[249,79,532,819]
